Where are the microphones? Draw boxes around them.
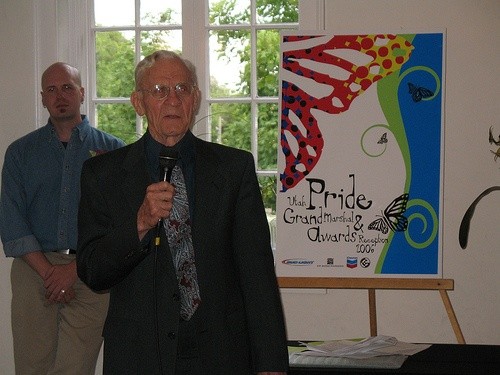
[155,148,178,246]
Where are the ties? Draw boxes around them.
[163,161,201,322]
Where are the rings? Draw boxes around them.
[60,289,65,293]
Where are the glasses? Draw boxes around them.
[138,83,193,97]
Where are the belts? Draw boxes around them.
[53,248,76,255]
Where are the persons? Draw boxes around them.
[0,63,126,375]
[76,50,289,375]
